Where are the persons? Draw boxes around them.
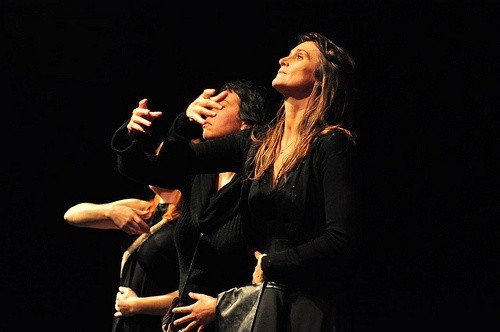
[170,33,362,332]
[112,80,277,331]
[63,139,201,331]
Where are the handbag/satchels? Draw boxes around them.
[213,283,324,332]
